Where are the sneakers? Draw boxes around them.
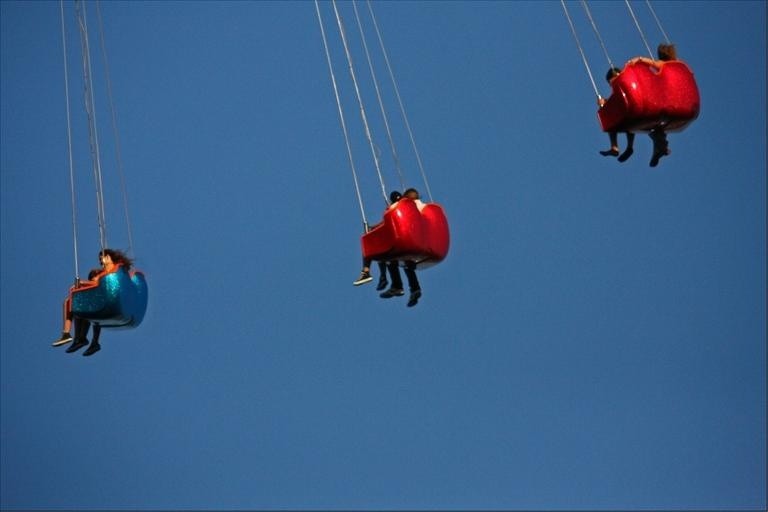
[353,271,374,287]
[376,278,422,306]
[600,145,667,167]
[52,332,100,356]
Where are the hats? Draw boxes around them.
[658,43,676,60]
[390,189,418,202]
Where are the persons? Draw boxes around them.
[379,185,428,308]
[52,246,116,350]
[648,44,678,168]
[599,66,635,162]
[351,188,403,291]
[64,248,136,357]
